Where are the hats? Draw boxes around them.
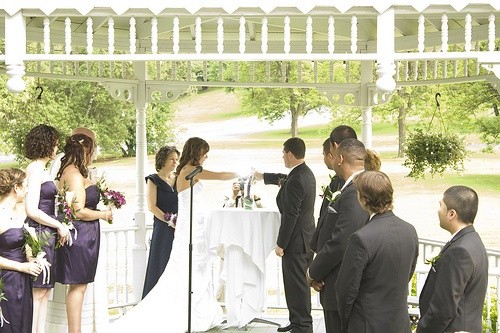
[71,128,96,142]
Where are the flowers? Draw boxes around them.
[277,176,286,187]
[395,122,468,181]
[54,179,80,250]
[22,222,57,285]
[100,188,126,224]
[163,212,177,227]
[325,189,342,206]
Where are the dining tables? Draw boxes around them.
[203,207,282,331]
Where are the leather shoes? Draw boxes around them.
[277,323,292,332]
[288,331,293,333]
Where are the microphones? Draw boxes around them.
[185,166,202,180]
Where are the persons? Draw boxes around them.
[142,145,181,298]
[305,125,419,333]
[0,124,113,333]
[216,181,265,301]
[114,137,241,333]
[415,186,488,333]
[254,137,316,333]
